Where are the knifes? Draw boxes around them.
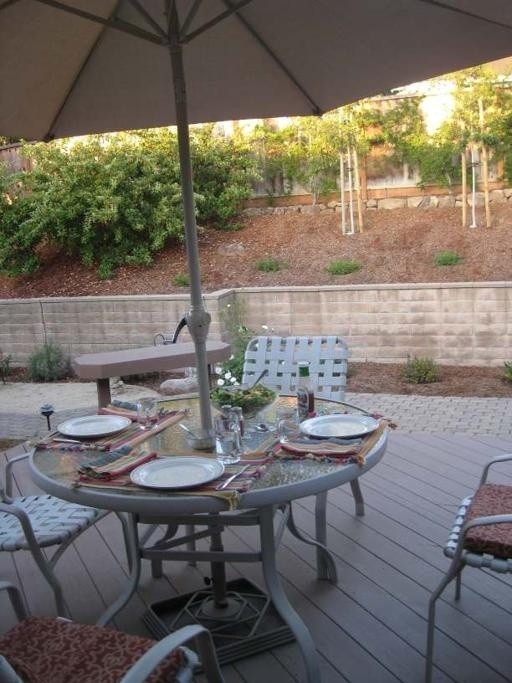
[52,436,107,446]
[217,461,251,490]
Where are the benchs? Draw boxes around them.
[72,338,232,415]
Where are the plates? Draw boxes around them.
[58,414,132,439]
[299,413,381,437]
[129,455,226,489]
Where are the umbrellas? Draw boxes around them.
[0,0,512,608]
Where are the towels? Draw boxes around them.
[77,445,157,485]
[271,436,363,458]
[96,400,160,426]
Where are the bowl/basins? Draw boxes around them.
[185,427,220,449]
[211,385,279,417]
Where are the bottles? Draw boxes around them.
[296,360,314,414]
[218,402,246,435]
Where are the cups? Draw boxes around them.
[276,406,301,442]
[216,430,241,465]
[137,397,158,431]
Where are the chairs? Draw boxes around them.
[0,452,142,629]
[184,335,364,580]
[0,581,226,682]
[425,452,511,683]
